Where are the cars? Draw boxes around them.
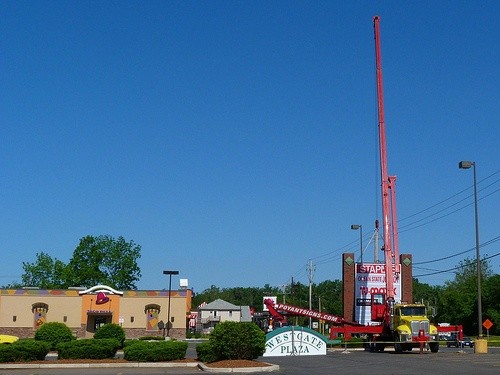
[447,334,475,348]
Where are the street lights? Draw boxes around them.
[459,162,487,354]
[163,270,179,336]
[351,225,363,262]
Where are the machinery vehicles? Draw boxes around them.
[265,18,439,354]
